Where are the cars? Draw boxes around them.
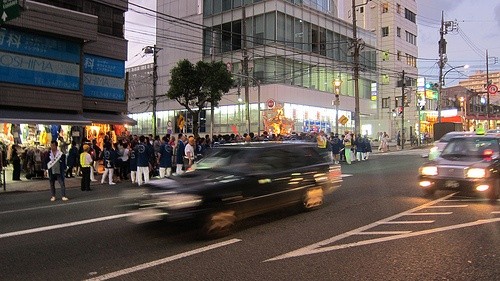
[417,129,500,198]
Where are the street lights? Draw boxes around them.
[437,65,470,122]
[401,83,431,150]
[333,78,344,134]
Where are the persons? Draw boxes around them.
[11,131,390,201]
[396,131,401,151]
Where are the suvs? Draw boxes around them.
[129,140,343,243]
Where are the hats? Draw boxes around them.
[83,145,90,151]
[188,137,195,143]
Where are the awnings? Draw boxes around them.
[0,108,136,125]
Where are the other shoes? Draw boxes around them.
[62,197,68,200]
[82,188,93,191]
[347,156,369,166]
[335,161,340,164]
[100,180,109,184]
[50,196,55,202]
[108,182,117,185]
[12,178,22,182]
[131,180,139,184]
[65,175,75,178]
